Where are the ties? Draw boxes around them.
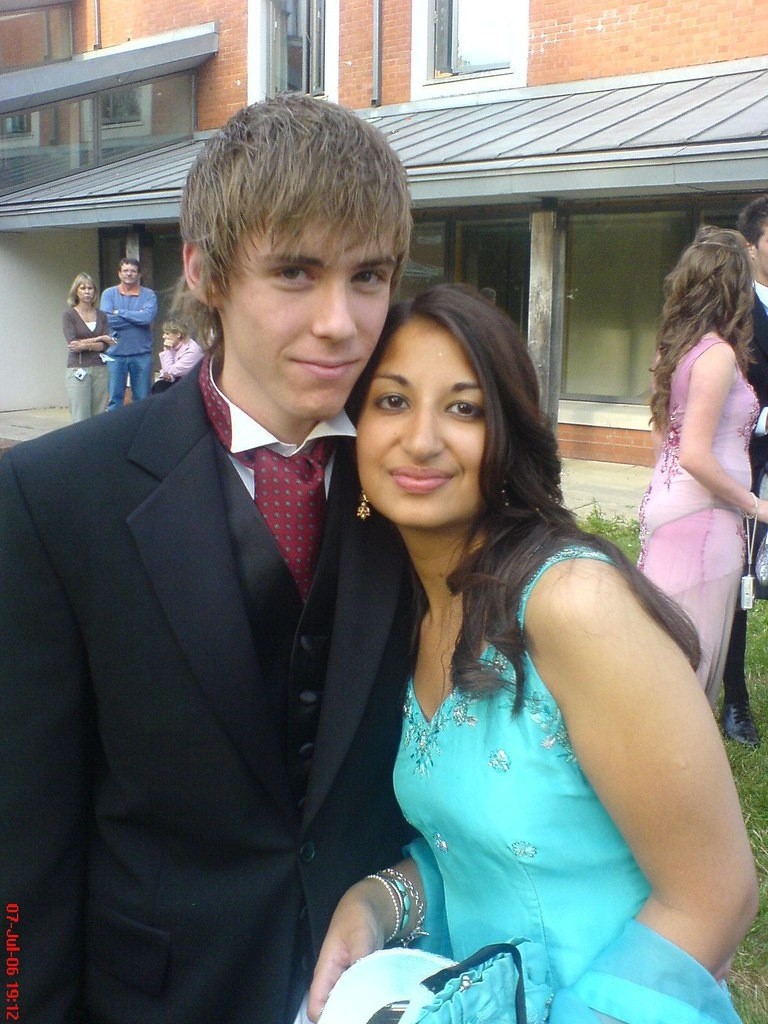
[200,340,337,605]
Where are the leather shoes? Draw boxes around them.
[722,699,761,749]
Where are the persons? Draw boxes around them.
[149,318,205,396]
[291,272,761,1024]
[0,93,412,1024]
[62,271,118,425]
[98,257,157,413]
[722,192,768,751]
[635,224,768,723]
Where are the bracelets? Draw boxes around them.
[361,868,430,950]
[745,492,758,520]
